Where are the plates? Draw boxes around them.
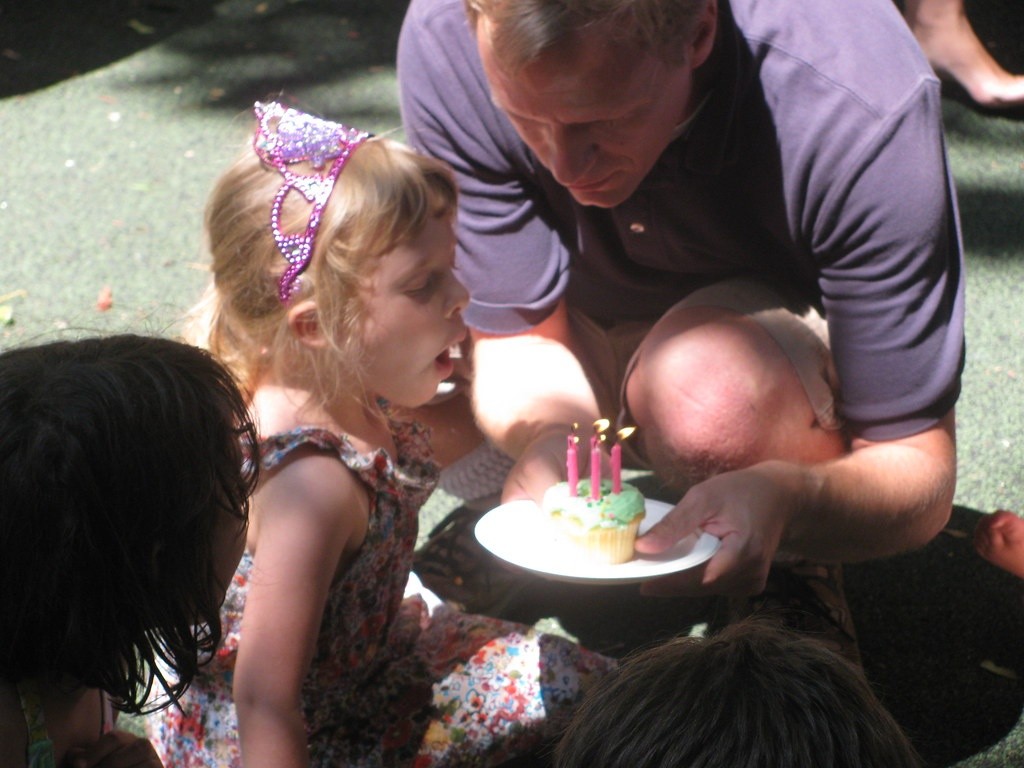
[474,497,722,586]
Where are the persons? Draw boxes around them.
[975,510,1024,570]
[903,0,1024,110]
[378,2,968,591]
[1,337,270,768]
[556,615,901,768]
[138,132,616,768]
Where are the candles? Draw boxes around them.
[591,435,606,499]
[609,427,636,492]
[591,419,610,449]
[568,422,581,483]
[567,436,580,497]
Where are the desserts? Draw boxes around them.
[544,478,645,567]
